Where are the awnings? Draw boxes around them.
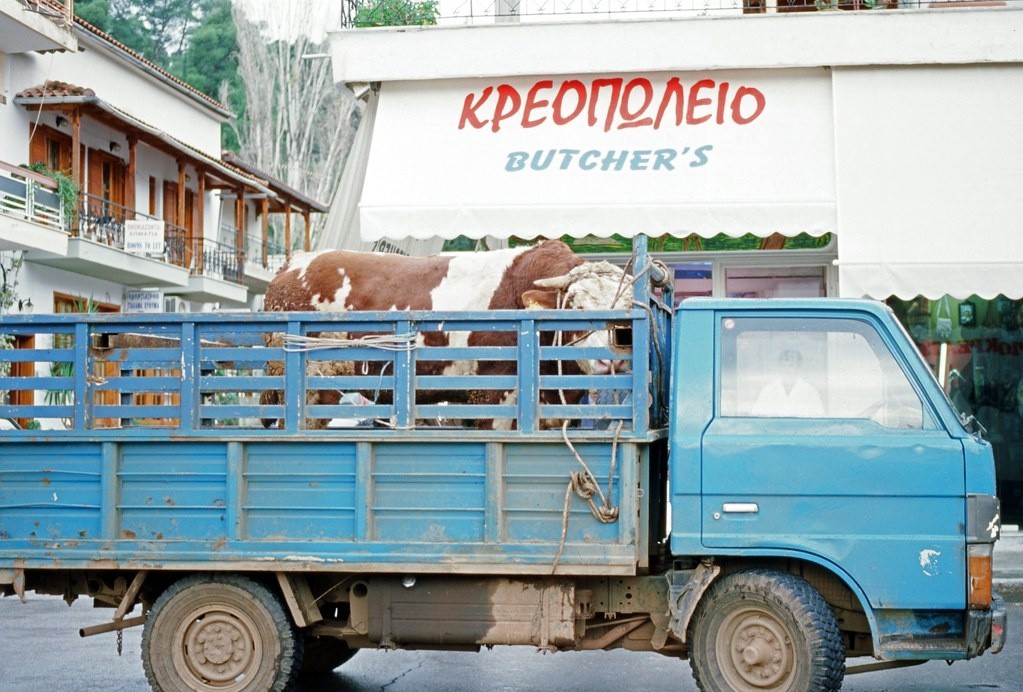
[833,64,1022,302]
[357,81,833,241]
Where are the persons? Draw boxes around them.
[580,389,597,427]
[754,352,822,417]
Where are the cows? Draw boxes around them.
[259,242,632,432]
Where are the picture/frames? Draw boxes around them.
[958,301,977,327]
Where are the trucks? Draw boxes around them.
[2,232,1007,691]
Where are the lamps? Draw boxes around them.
[352,82,370,100]
[109,141,121,152]
[56,115,69,127]
[18,298,33,312]
